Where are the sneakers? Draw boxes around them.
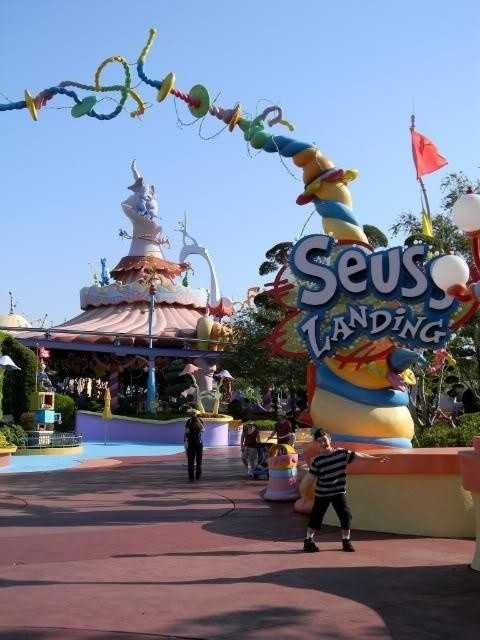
[341,538,355,552]
[304,537,320,552]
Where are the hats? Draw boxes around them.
[246,420,254,425]
[276,411,287,416]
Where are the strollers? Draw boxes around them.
[249,437,285,481]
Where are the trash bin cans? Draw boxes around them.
[263,443,300,500]
[229,420,244,446]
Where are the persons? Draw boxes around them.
[241,420,260,477]
[184,409,206,481]
[300,427,391,552]
[268,411,299,447]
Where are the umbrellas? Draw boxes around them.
[103,387,112,445]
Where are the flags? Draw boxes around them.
[412,130,449,180]
[422,214,434,259]
